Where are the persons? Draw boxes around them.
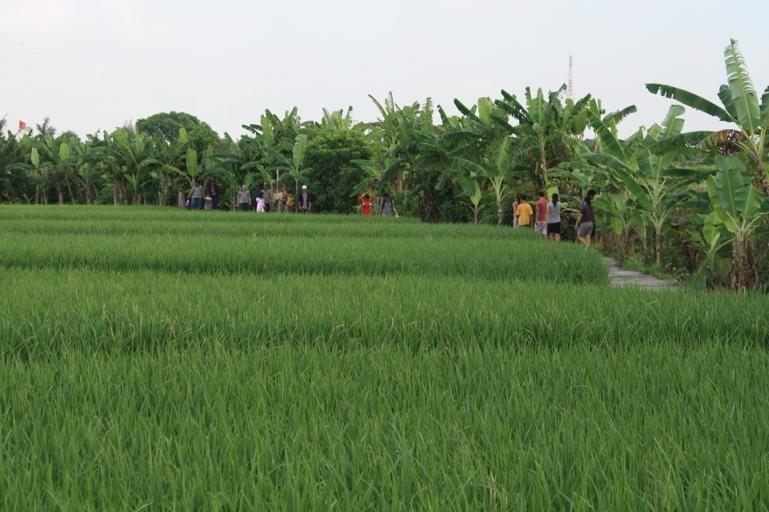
[181,179,310,215]
[511,193,521,228]
[544,193,561,242]
[574,198,594,252]
[514,194,533,227]
[357,193,374,217]
[361,189,373,207]
[377,192,398,217]
[534,191,547,236]
[579,189,596,239]
